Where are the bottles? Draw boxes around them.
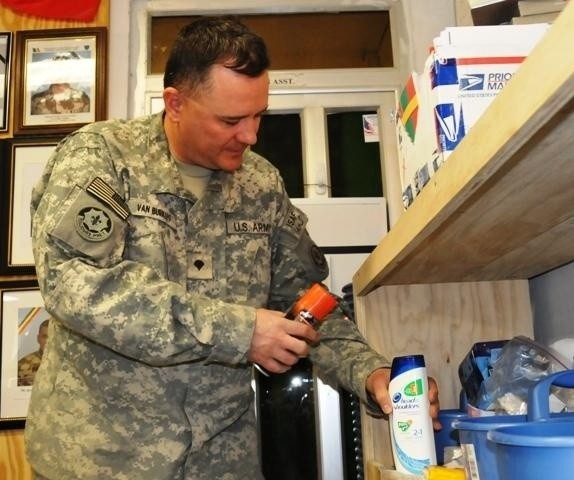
[251,283,335,378]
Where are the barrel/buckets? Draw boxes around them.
[434,370,574,480]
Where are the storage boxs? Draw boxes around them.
[388,18,554,214]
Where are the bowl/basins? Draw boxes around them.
[434,371,573,480]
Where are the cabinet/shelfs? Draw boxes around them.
[353,0,570,478]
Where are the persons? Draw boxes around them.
[22,12,442,480]
[18,319,50,387]
[31,52,89,114]
